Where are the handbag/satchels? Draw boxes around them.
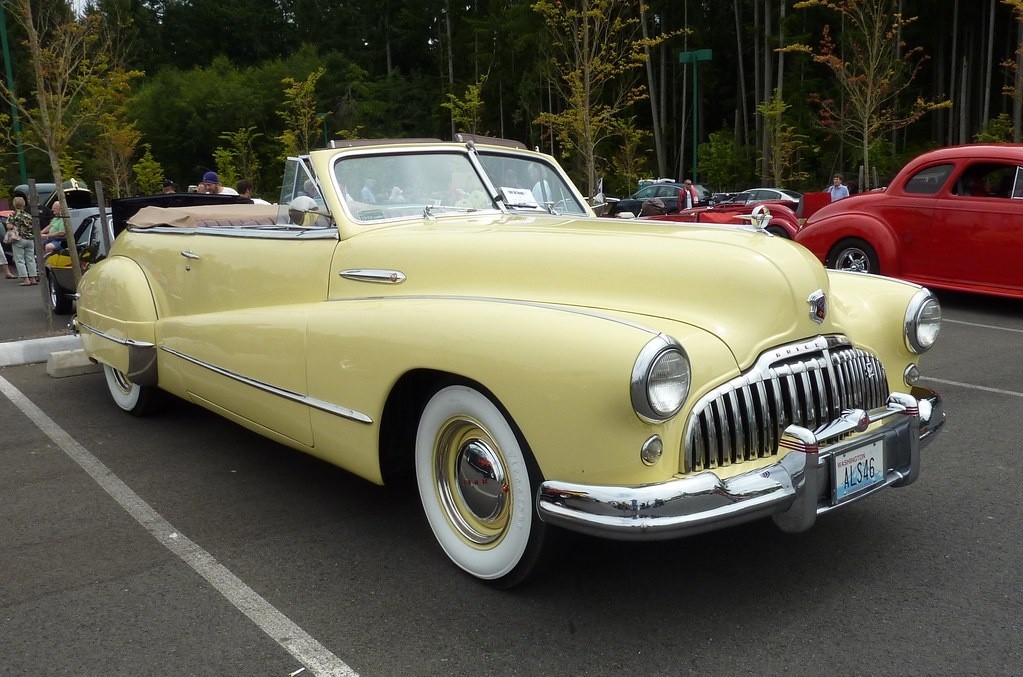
[3,212,20,244]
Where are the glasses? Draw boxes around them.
[685,183,692,185]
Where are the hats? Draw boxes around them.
[203,172,220,184]
[163,180,178,187]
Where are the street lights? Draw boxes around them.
[679,49,712,184]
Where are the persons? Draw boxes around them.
[362,179,376,203]
[0,243,18,279]
[827,174,850,202]
[389,187,406,203]
[527,162,551,209]
[278,179,316,204]
[162,171,272,205]
[678,179,699,213]
[41,201,66,253]
[6,197,38,286]
[504,169,523,189]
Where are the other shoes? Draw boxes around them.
[6,274,18,279]
[19,282,31,286]
[31,282,38,285]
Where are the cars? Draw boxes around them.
[720,187,803,203]
[0,178,92,263]
[274,191,323,205]
[793,142,1023,300]
[37,193,255,315]
[609,183,717,218]
[68,133,946,587]
[592,199,799,241]
